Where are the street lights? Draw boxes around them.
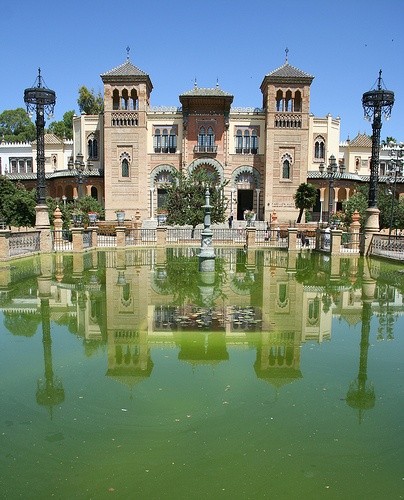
[67,154,93,205]
[70,274,89,314]
[311,275,342,316]
[319,156,348,234]
